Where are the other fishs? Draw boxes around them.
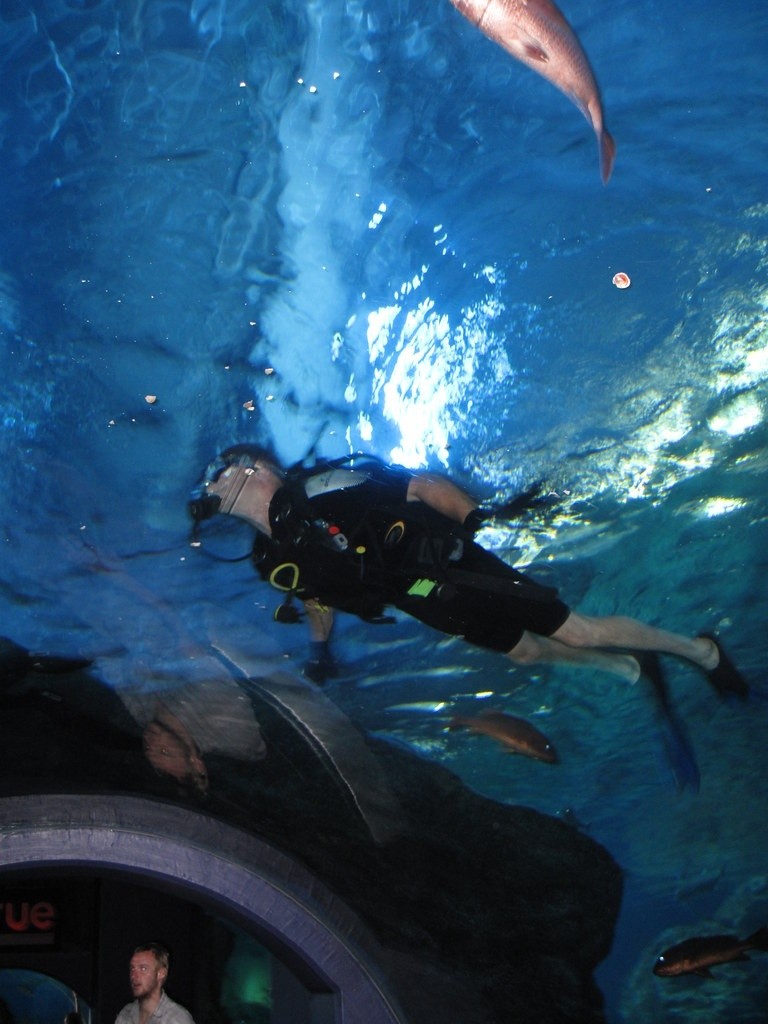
[651,928,768,978]
[448,711,561,767]
[448,0,619,190]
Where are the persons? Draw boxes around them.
[205,444,750,718]
[74,541,266,798]
[114,943,196,1024]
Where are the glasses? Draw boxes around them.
[197,459,241,495]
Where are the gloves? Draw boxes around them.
[463,487,549,537]
[301,641,337,685]
